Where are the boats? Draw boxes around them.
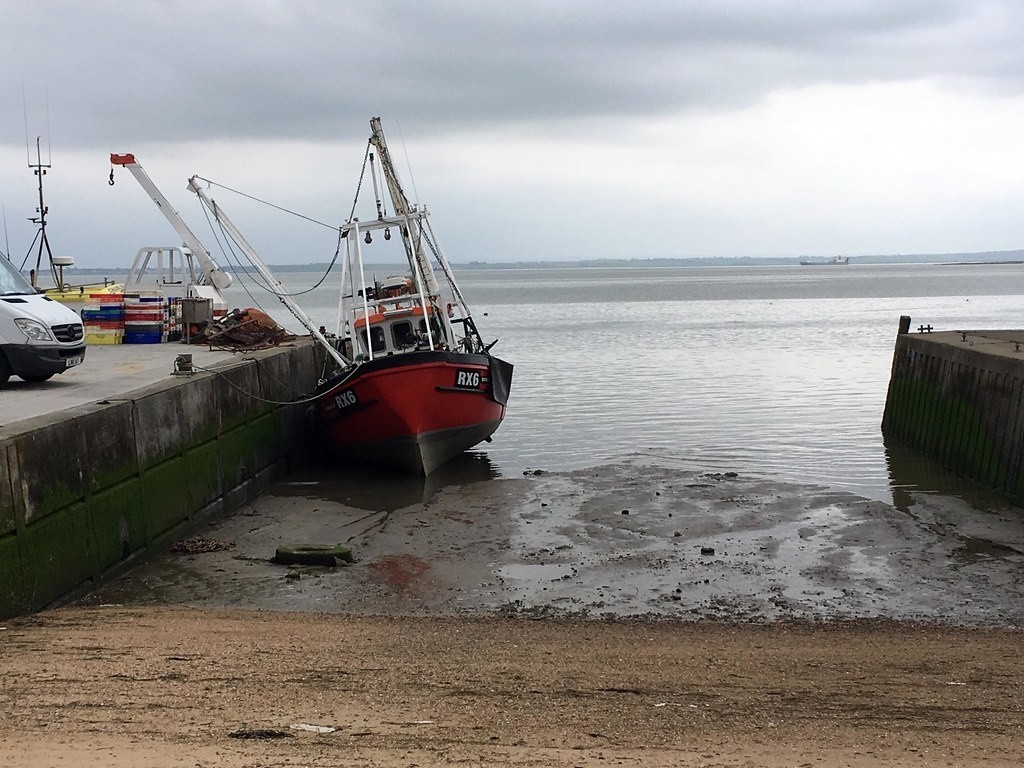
[187,116,514,475]
[800,254,849,266]
[103,153,233,323]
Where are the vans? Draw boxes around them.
[0,251,86,388]
